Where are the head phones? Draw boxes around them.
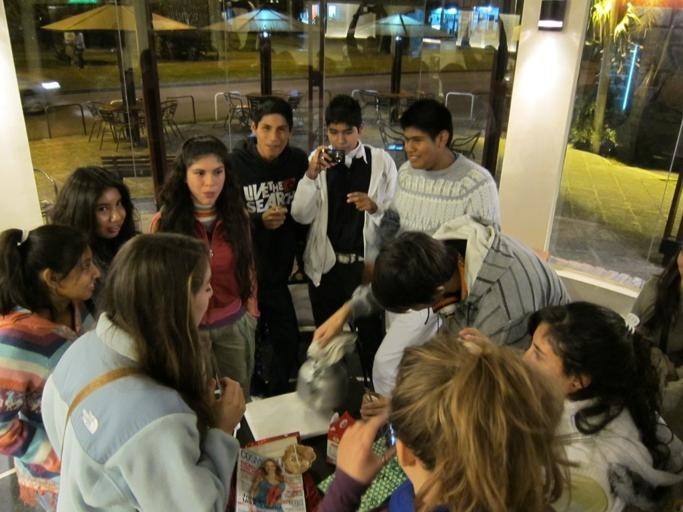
[431,253,468,319]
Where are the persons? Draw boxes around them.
[224,93,309,394]
[249,458,286,512]
[366,94,500,245]
[289,90,400,329]
[0,218,246,512]
[43,164,146,310]
[148,133,262,407]
[315,216,683,512]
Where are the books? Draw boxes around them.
[235,447,306,512]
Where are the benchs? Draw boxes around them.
[104,155,178,184]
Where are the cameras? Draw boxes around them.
[325,149,345,163]
[384,422,396,447]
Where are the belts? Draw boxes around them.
[335,252,365,264]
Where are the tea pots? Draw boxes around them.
[296,356,348,413]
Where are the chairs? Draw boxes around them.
[33,168,58,225]
[83,98,185,153]
[359,86,481,161]
[222,89,306,131]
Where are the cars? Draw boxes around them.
[16,72,62,114]
